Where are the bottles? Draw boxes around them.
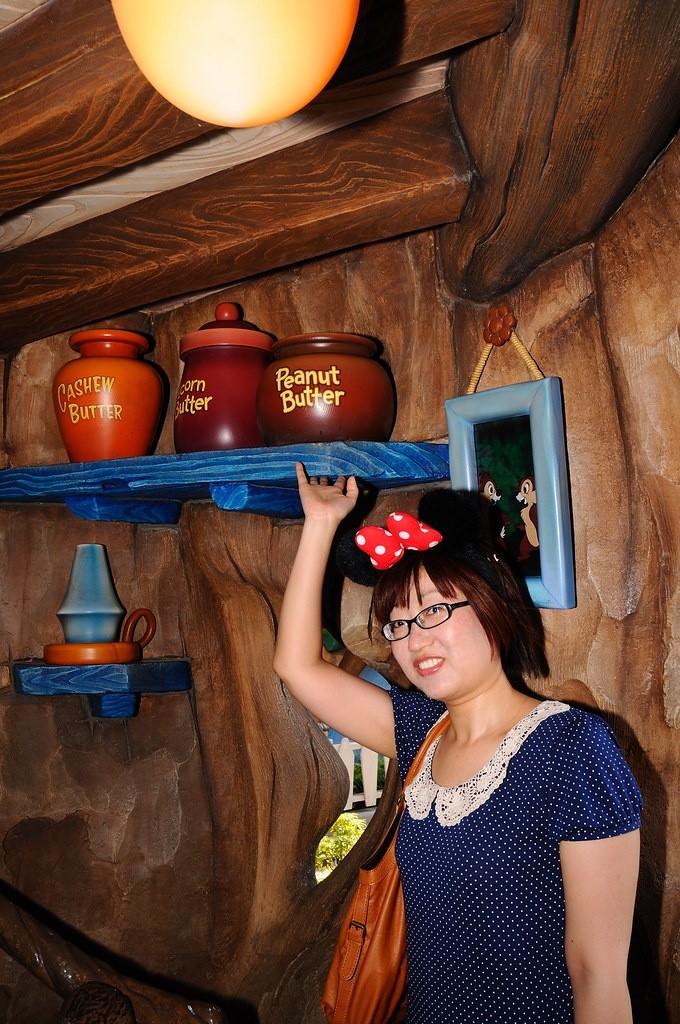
[255,334,394,446]
[175,303,274,455]
[53,329,163,464]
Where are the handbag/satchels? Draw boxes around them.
[319,710,451,1024]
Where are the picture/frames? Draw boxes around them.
[444,377,575,610]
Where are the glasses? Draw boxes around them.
[380,600,471,641]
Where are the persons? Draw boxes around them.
[273,455,645,1024]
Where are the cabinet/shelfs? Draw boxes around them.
[0,443,450,719]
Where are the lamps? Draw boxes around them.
[111,0,359,128]
